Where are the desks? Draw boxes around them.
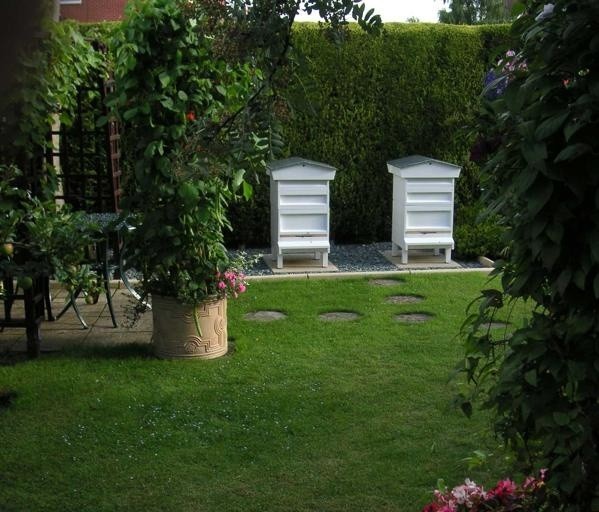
[54,212,152,330]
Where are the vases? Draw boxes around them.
[150,288,227,360]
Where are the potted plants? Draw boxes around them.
[107,0,383,300]
